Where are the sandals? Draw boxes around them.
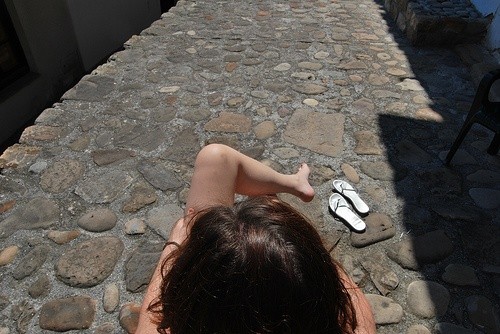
[328,192,367,232]
[332,180,370,214]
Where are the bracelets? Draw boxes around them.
[161,240,183,252]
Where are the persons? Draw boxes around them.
[133,140,375,334]
